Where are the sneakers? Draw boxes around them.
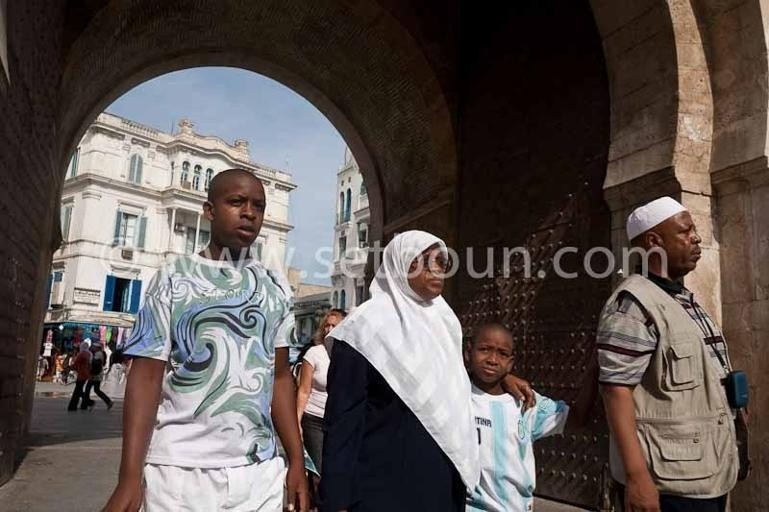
[67,401,114,414]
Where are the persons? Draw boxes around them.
[100,168,312,512]
[464,319,601,511]
[313,231,536,512]
[594,197,751,512]
[295,306,347,481]
[38,339,130,412]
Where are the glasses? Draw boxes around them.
[412,257,452,269]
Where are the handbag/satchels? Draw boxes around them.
[728,372,749,410]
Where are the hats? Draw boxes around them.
[625,195,689,242]
